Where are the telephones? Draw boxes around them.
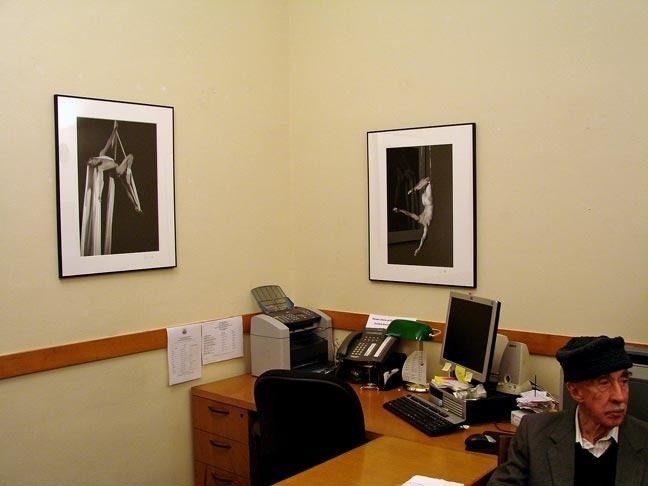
[336,328,399,364]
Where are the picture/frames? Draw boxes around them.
[366,122,478,289]
[52,94,178,280]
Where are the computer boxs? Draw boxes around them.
[428,381,521,424]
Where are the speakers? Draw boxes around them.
[489,334,509,382]
[496,341,533,395]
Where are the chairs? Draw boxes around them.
[253,369,367,477]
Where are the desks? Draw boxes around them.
[274,436,498,485]
[191,373,518,458]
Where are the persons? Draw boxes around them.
[391,176,434,257]
[86,119,142,216]
[483,332,647,486]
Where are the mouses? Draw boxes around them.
[464,433,497,449]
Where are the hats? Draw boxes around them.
[555,334,633,383]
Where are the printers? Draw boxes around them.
[250,285,337,377]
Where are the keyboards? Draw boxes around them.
[382,393,465,436]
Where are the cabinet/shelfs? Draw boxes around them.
[191,393,255,484]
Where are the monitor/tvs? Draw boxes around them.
[440,290,501,383]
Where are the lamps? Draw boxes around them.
[384,319,433,392]
